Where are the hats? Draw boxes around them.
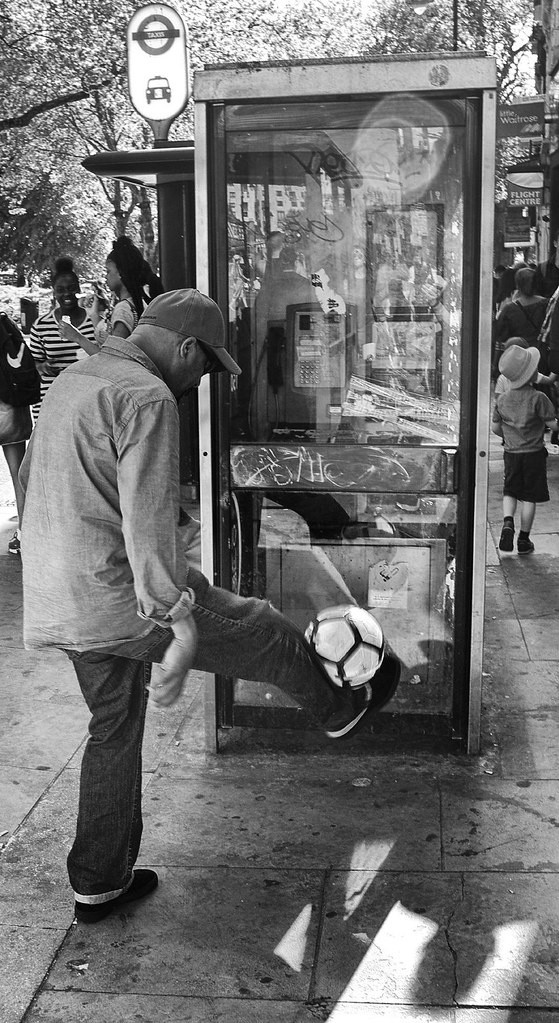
[137,288,242,375]
[498,344,541,390]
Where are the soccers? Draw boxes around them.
[302,607,386,688]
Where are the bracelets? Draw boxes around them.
[433,300,439,307]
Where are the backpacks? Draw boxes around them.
[0,311,43,408]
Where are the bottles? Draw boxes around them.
[61,316,71,341]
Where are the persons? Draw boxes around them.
[0,312,33,555]
[491,344,559,555]
[16,289,401,925]
[230,231,312,308]
[58,236,164,356]
[373,263,446,334]
[493,229,559,445]
[29,258,101,433]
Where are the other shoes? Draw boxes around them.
[499,525,515,551]
[71,868,159,923]
[6,531,21,554]
[542,440,559,454]
[323,656,401,745]
[516,539,535,555]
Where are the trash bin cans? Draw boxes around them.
[19,297,38,335]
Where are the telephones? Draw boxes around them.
[265,301,360,424]
